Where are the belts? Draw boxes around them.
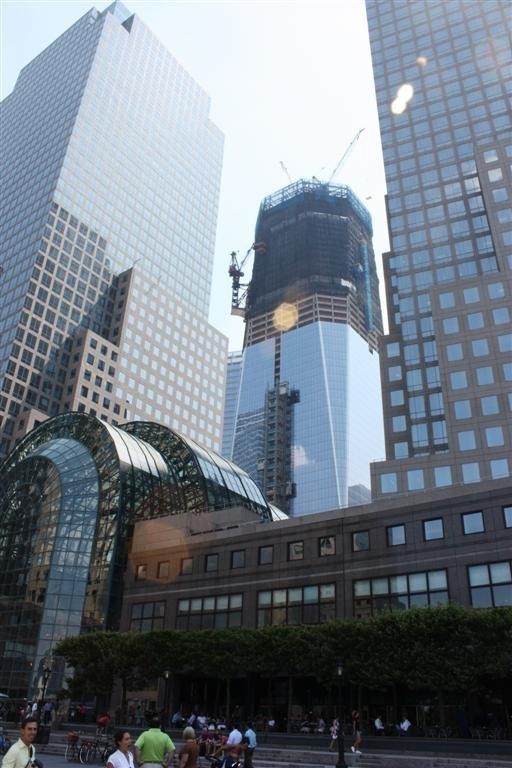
[144,761,161,763]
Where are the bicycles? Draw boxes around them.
[64,727,117,766]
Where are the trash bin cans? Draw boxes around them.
[34,726,51,744]
